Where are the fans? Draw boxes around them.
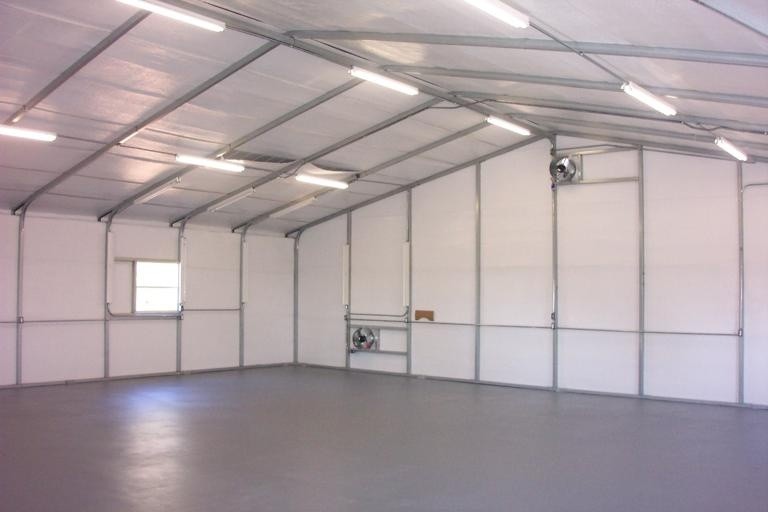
[352,326,375,350]
[550,155,576,183]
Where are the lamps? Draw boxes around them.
[294,170,351,189]
[468,1,531,30]
[709,134,747,162]
[343,62,421,98]
[1,121,59,145]
[621,78,679,119]
[119,0,229,34]
[483,113,532,137]
[171,150,246,174]
